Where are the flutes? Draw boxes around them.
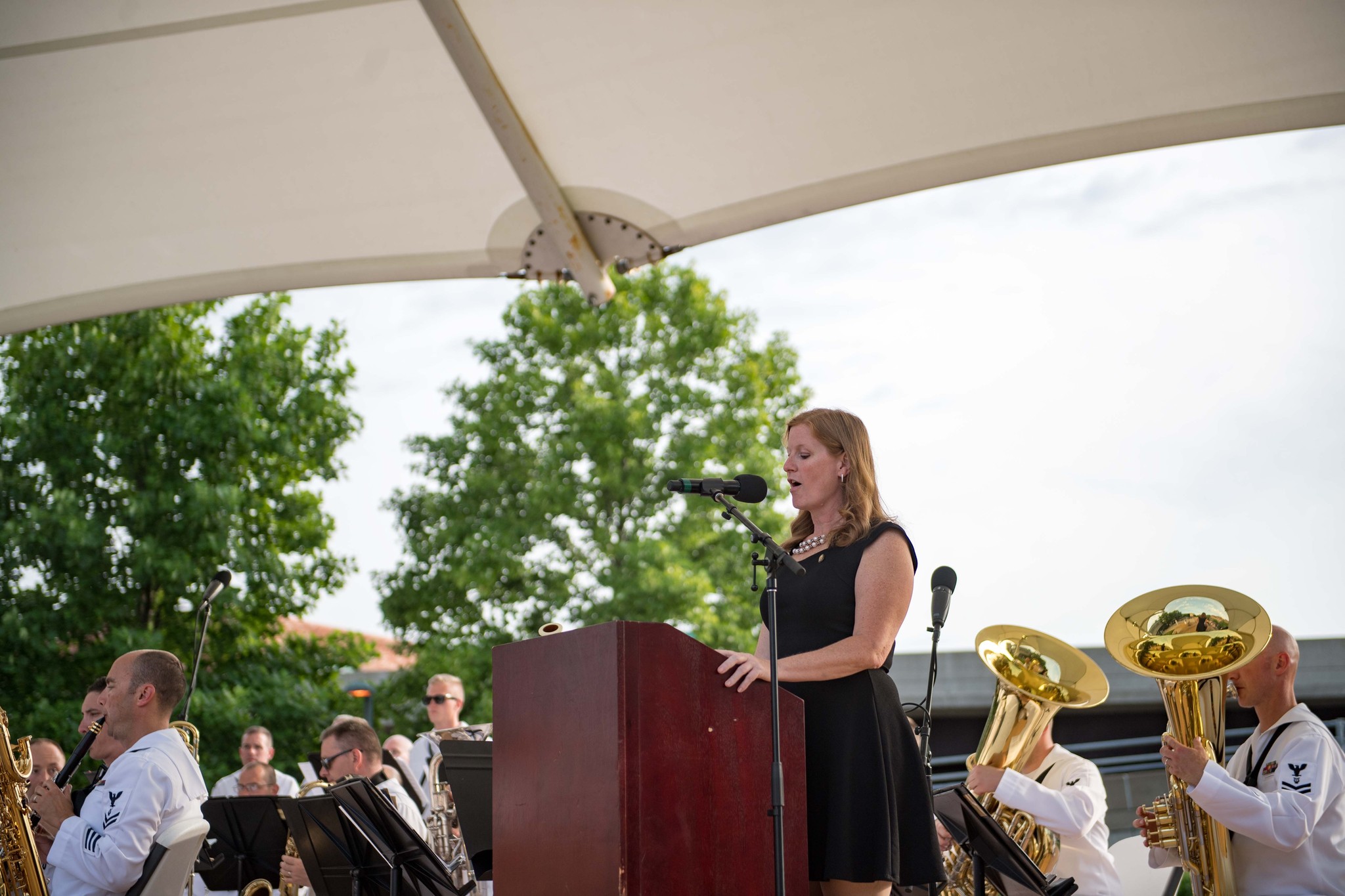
[30,715,105,832]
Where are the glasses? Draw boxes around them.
[320,748,364,771]
[232,783,276,792]
[421,694,463,705]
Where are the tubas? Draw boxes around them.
[935,624,1110,896]
[415,723,493,896]
[1103,582,1273,896]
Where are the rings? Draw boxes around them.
[1165,759,1169,764]
[288,871,291,877]
[33,795,39,801]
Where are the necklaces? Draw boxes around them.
[792,535,829,554]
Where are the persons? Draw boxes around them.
[929,714,1130,896]
[1132,625,1345,896]
[717,409,949,896]
[197,674,494,896]
[0,648,211,896]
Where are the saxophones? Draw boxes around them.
[239,779,336,896]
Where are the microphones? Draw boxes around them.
[198,569,232,611]
[930,565,957,639]
[667,474,767,503]
